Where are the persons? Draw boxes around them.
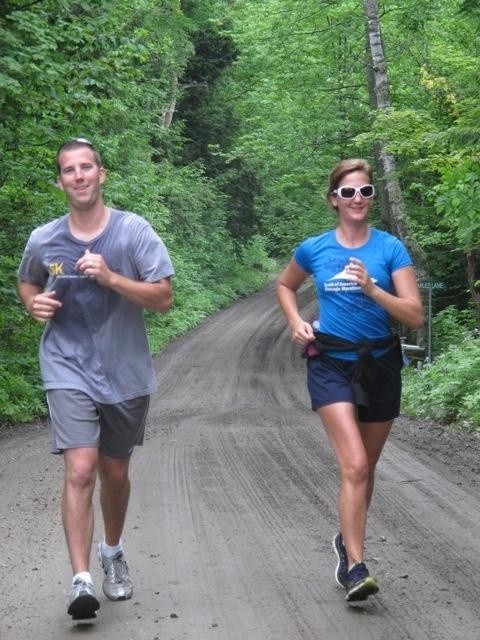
[275,158,425,601]
[16,137,175,621]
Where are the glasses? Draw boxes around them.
[76,138,95,151]
[333,184,375,200]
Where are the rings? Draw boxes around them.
[87,261,93,268]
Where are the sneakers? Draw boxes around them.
[344,558,379,602]
[332,532,349,590]
[97,542,133,601]
[66,576,100,620]
